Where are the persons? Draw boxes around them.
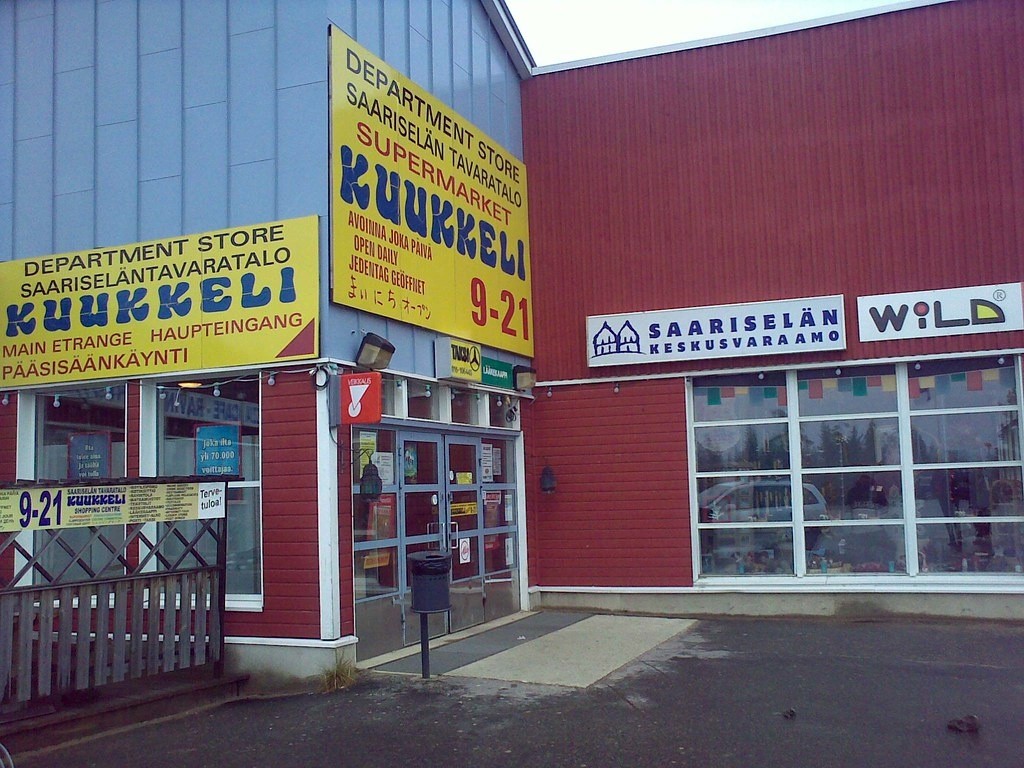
[927,468,993,550]
[844,474,889,509]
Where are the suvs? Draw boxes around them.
[696,479,832,550]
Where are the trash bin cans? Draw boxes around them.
[407,551,452,615]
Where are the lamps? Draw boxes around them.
[340,440,383,503]
[2,393,9,405]
[355,330,395,369]
[178,383,202,388]
[512,365,537,391]
[527,454,557,493]
